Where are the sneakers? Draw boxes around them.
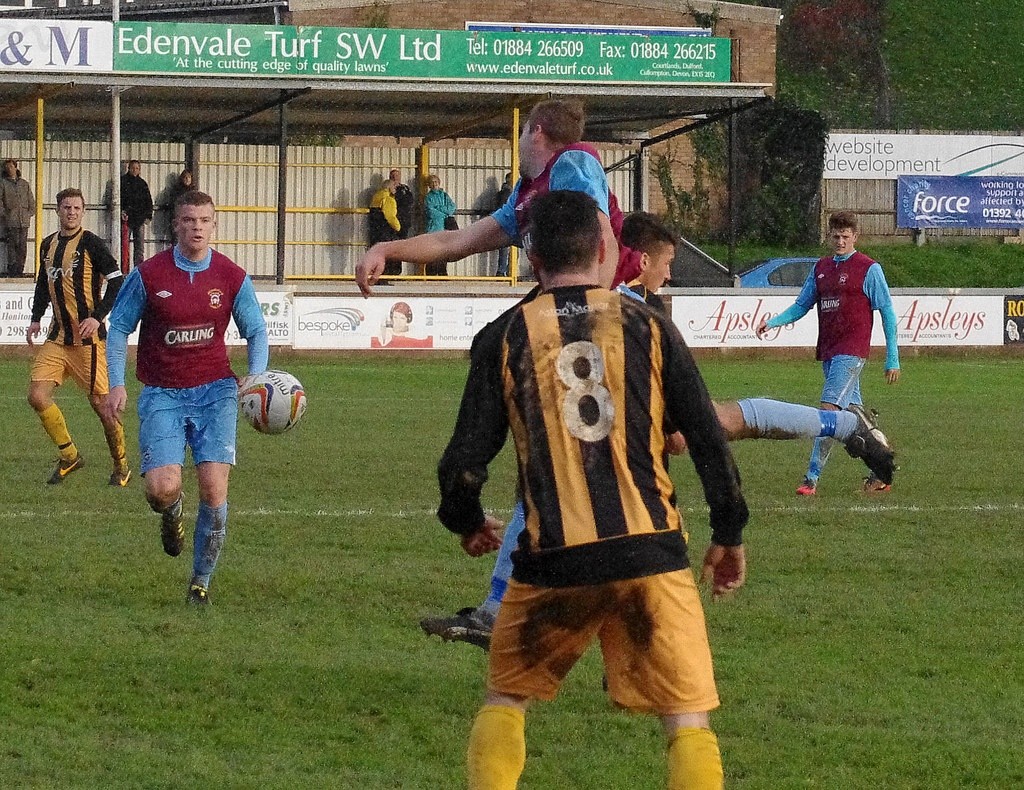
[108,468,133,486]
[185,583,212,607]
[160,490,184,557]
[418,607,495,652]
[841,404,895,484]
[795,473,817,495]
[49,450,86,483]
[861,471,890,492]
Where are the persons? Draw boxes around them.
[26,188,133,486]
[370,178,401,286]
[106,189,269,606]
[354,97,893,694]
[0,159,35,278]
[496,173,519,283]
[424,174,457,280]
[170,170,196,246]
[120,160,153,269]
[436,189,749,790]
[390,169,413,280]
[755,207,899,496]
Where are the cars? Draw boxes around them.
[739,257,824,287]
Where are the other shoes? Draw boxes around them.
[7,273,27,278]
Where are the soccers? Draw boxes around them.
[240,370,307,436]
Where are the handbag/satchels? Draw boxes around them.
[444,215,460,231]
